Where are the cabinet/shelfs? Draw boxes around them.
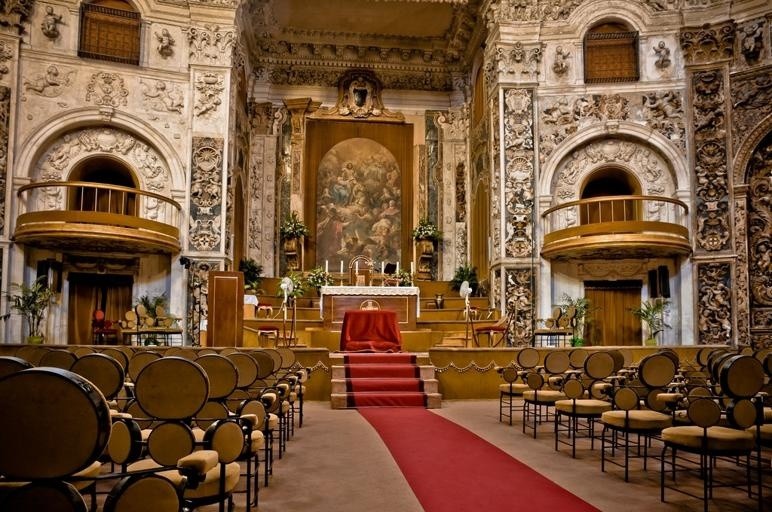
[339,312,402,353]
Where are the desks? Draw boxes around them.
[317,285,421,322]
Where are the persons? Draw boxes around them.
[468,266,479,297]
[24,6,224,219]
[486,0,772,347]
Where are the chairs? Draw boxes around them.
[91,306,186,346]
[493,348,771,511]
[475,304,577,348]
[0,336,321,512]
[358,298,382,310]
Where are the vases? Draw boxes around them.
[435,294,443,309]
[421,240,434,254]
[282,236,298,254]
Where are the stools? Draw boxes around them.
[257,325,280,348]
[255,302,274,319]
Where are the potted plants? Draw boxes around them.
[302,265,332,298]
[274,273,300,310]
[627,298,671,349]
[559,293,590,348]
[6,280,56,347]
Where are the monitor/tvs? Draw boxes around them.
[384,264,396,276]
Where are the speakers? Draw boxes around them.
[36,258,62,293]
[658,267,670,298]
[647,269,658,298]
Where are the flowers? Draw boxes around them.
[411,221,441,240]
[277,211,307,240]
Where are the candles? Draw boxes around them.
[321,258,416,277]
